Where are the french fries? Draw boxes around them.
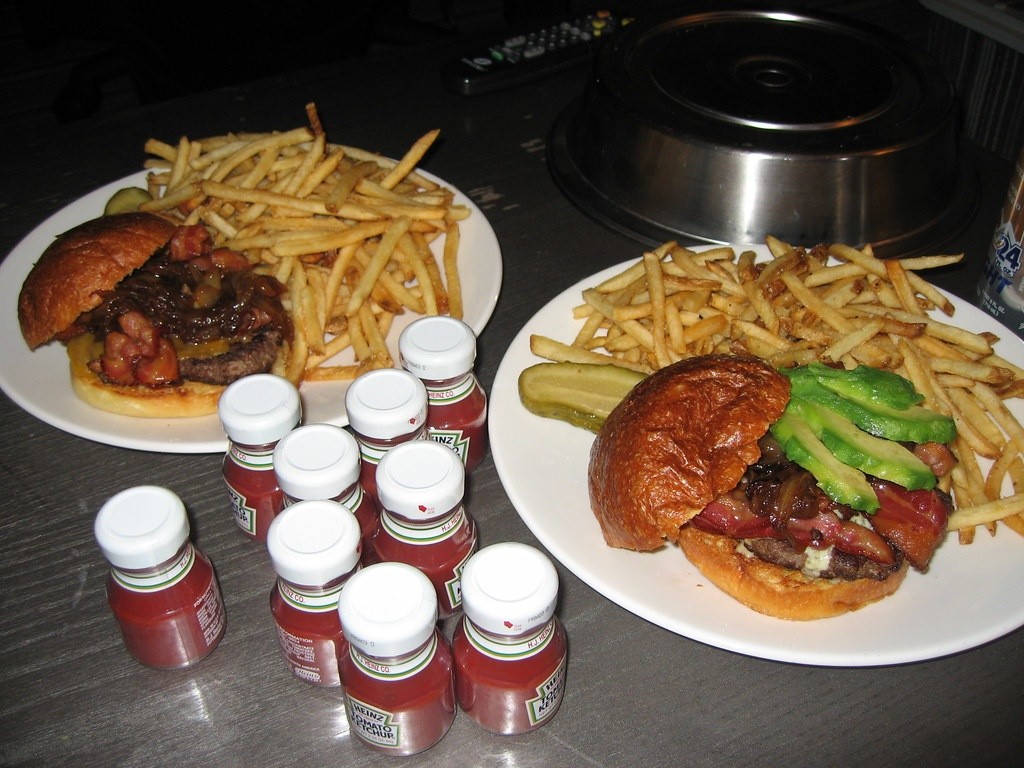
[531,235,1024,545]
[144,103,471,381]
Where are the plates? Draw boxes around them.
[1,159,502,454]
[488,244,1024,665]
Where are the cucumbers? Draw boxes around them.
[517,362,649,434]
[104,187,152,215]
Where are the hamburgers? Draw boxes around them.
[589,353,960,621]
[18,211,308,419]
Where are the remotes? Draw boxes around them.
[439,10,640,96]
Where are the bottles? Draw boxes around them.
[451,542,567,736]
[94,485,227,671]
[974,152,1024,342]
[374,440,478,619]
[336,562,456,757]
[216,374,302,540]
[345,368,433,518]
[398,315,488,475]
[272,423,380,570]
[267,499,362,688]
